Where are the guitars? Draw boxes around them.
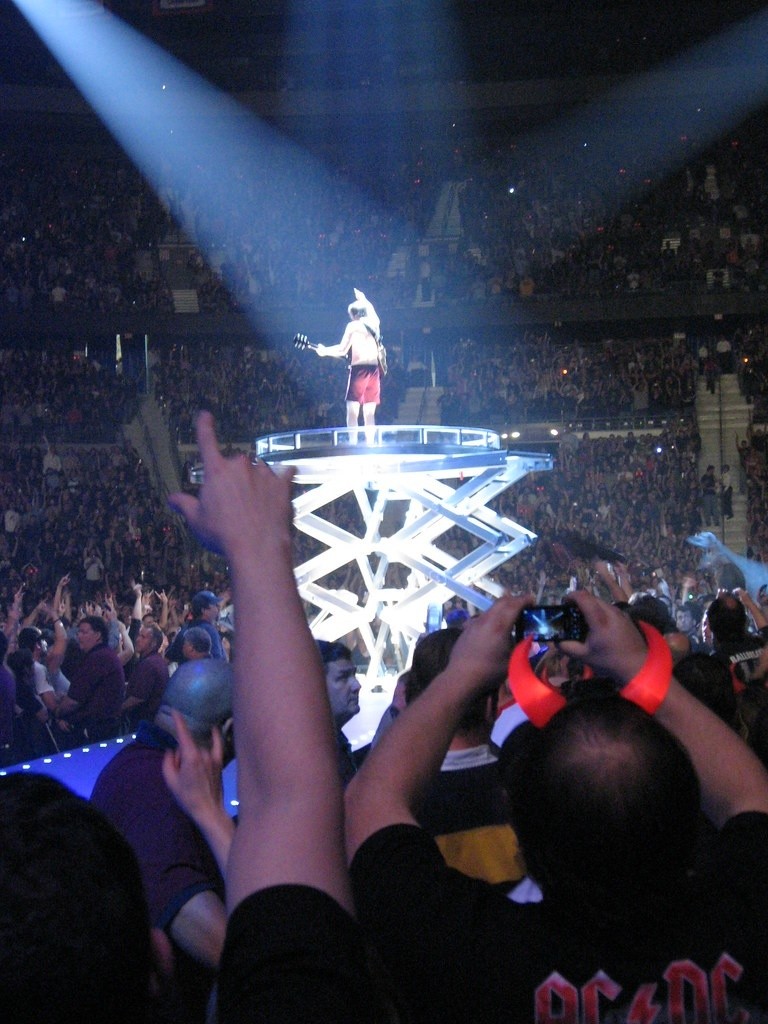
[293,333,387,379]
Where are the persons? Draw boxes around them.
[0,150,768,1023]
[316,286,381,446]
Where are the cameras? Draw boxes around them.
[515,603,589,643]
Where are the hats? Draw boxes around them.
[446,609,469,623]
[18,627,49,645]
[192,591,224,609]
